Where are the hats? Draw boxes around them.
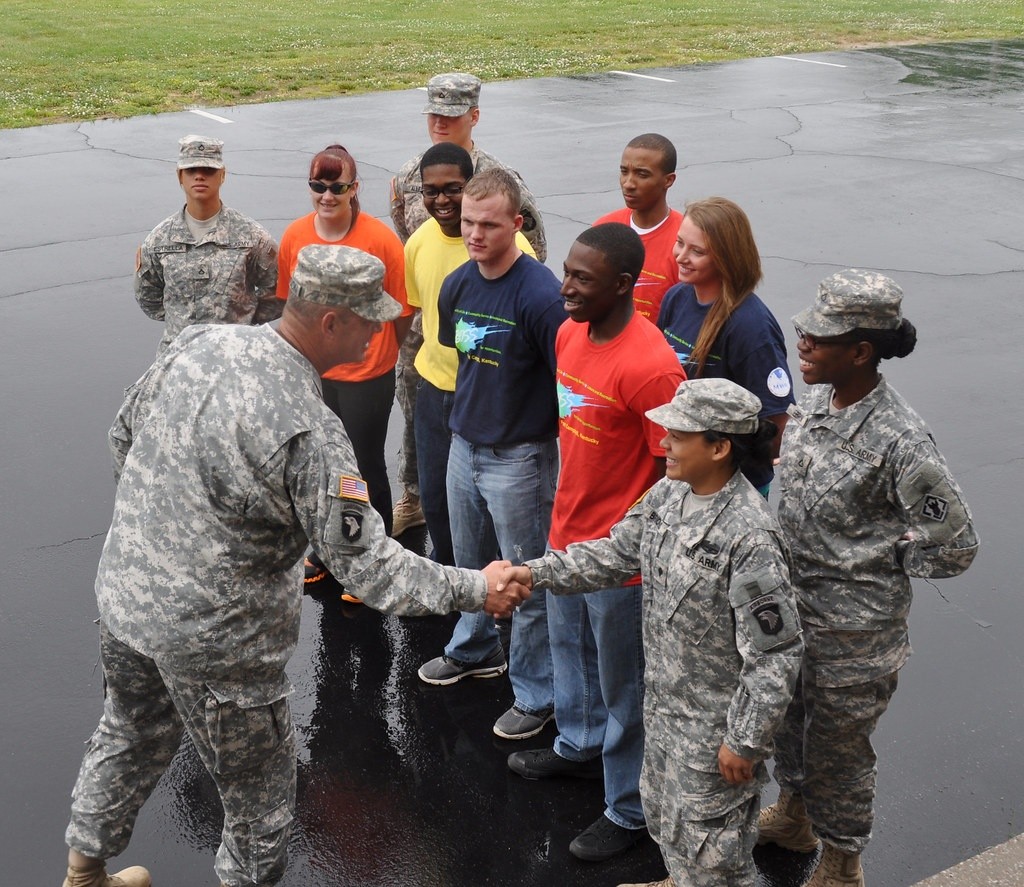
[176,135,224,169]
[791,270,903,337]
[644,378,762,434]
[421,73,484,117]
[289,244,403,321]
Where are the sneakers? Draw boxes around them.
[301,551,328,583]
[569,815,646,861]
[617,875,675,887]
[493,703,554,739]
[417,640,507,685]
[757,791,820,852]
[801,841,864,887]
[392,491,426,539]
[341,588,361,603]
[508,747,581,781]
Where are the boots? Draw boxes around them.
[60,849,151,887]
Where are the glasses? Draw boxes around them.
[419,177,470,199]
[795,327,849,349]
[308,177,355,195]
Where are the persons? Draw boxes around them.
[507,222,688,863]
[275,145,415,604]
[591,133,685,326]
[486,379,805,887]
[62,245,531,887]
[418,169,573,739]
[758,269,980,887]
[404,142,536,566]
[134,136,286,361]
[657,197,795,498]
[390,72,547,538]
